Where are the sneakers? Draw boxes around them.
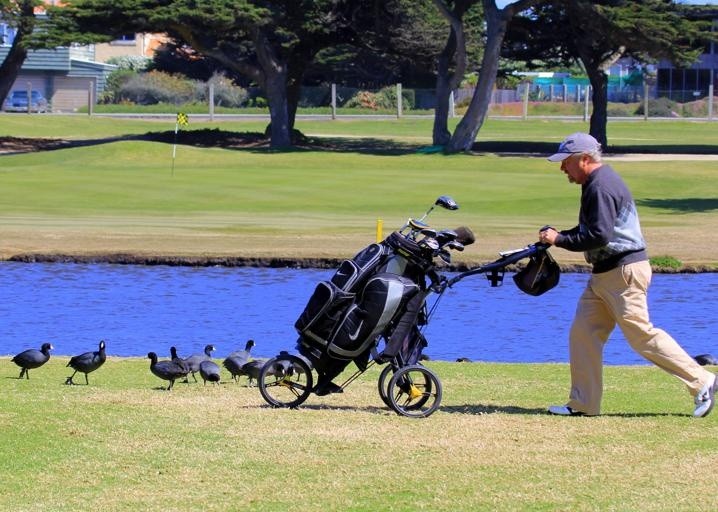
[548,405,600,416]
[693,372,718,417]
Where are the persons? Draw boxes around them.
[539,132,718,419]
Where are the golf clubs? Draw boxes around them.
[399,196,464,262]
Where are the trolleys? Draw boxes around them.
[254,219,558,419]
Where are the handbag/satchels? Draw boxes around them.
[513,251,559,295]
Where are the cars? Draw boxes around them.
[4,89,48,114]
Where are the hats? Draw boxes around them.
[548,133,598,162]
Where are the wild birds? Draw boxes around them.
[10,342,54,380]
[65,340,106,385]
[143,338,313,391]
